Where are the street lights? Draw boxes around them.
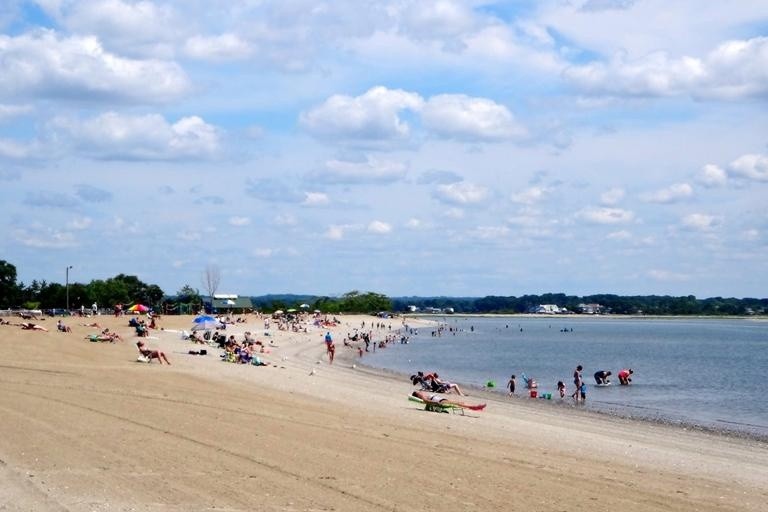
[66,265,73,317]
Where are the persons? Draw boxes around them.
[136,341,171,367]
[593,371,611,385]
[113,301,121,317]
[0,318,15,326]
[90,302,97,316]
[556,381,565,399]
[571,365,583,401]
[505,374,515,398]
[22,322,48,333]
[101,331,122,341]
[617,368,633,385]
[19,312,38,321]
[56,320,72,334]
[432,373,466,397]
[188,305,479,372]
[578,381,585,401]
[412,390,486,411]
[83,322,100,328]
[79,303,84,319]
[130,311,158,336]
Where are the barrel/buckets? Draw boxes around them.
[547,392,552,399]
[531,390,537,398]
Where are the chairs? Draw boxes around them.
[136,342,153,364]
[412,373,450,392]
[409,393,465,415]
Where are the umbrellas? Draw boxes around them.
[127,303,150,315]
